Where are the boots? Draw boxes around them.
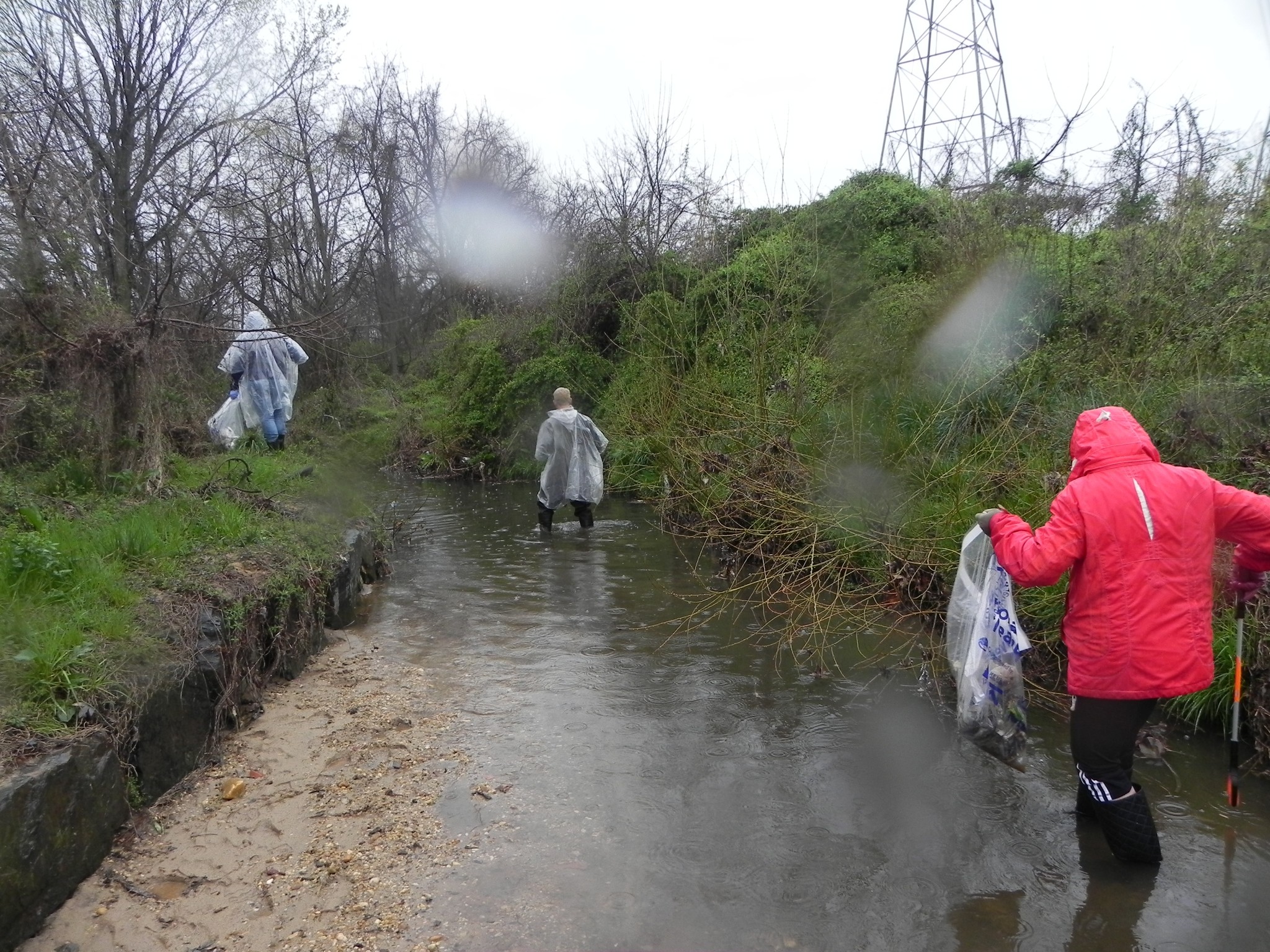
[539,512,553,532]
[278,434,285,451]
[579,514,593,527]
[1088,783,1163,864]
[265,436,279,450]
[1077,779,1096,819]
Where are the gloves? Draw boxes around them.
[230,390,238,400]
[975,508,1002,537]
[1230,564,1266,603]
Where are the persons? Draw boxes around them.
[217,311,308,455]
[535,387,609,531]
[976,406,1270,866]
